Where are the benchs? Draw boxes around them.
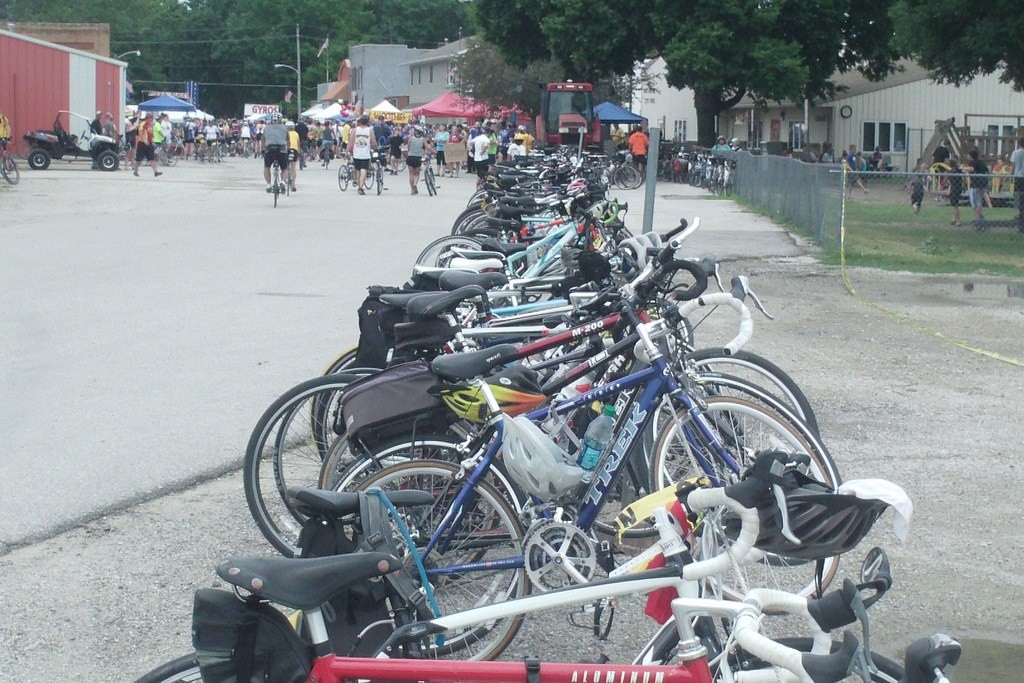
[862,154,899,178]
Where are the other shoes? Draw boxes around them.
[92,166,99,169]
[434,168,459,178]
[303,162,306,167]
[321,163,325,166]
[951,219,962,226]
[290,183,296,192]
[133,172,139,176]
[266,183,271,193]
[280,181,287,193]
[411,185,418,195]
[155,172,162,176]
[358,188,365,195]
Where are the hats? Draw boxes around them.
[518,125,524,130]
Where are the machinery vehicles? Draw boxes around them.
[534,78,604,154]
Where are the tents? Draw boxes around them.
[302,91,531,123]
[138,94,197,112]
[582,101,642,124]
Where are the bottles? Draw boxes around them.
[540,369,598,434]
[572,405,616,484]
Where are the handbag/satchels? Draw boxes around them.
[354,285,408,368]
[190,586,312,683]
[330,357,455,455]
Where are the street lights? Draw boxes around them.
[272,64,303,120]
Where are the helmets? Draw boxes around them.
[724,470,891,565]
[440,362,547,424]
[270,112,283,121]
[414,125,424,134]
[577,250,611,280]
[513,132,524,140]
[501,412,587,500]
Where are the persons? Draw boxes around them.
[261,112,292,194]
[124,109,534,180]
[712,135,742,151]
[407,125,430,195]
[962,149,989,228]
[810,147,866,171]
[1009,137,1024,218]
[946,159,962,227]
[612,123,648,170]
[846,144,871,199]
[90,110,117,170]
[284,121,300,192]
[0,112,11,153]
[993,158,1012,183]
[932,139,951,173]
[871,147,882,164]
[911,175,927,214]
[345,114,378,195]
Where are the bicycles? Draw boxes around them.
[420,150,440,197]
[0,135,21,184]
[128,136,961,683]
